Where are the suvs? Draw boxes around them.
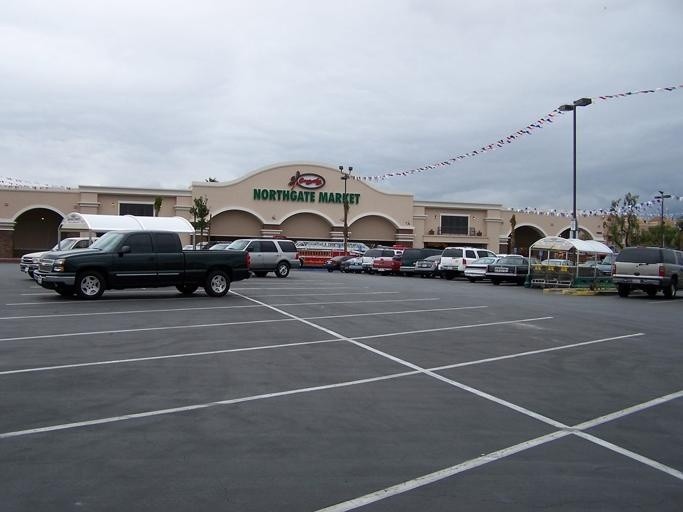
[226,239,303,277]
[399,248,443,276]
[19,237,99,279]
[437,246,501,283]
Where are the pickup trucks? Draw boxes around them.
[32,230,250,300]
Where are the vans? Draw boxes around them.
[611,247,683,299]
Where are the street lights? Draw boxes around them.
[654,191,671,249]
[559,98,591,266]
[338,165,353,255]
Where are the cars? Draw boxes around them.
[340,248,403,275]
[591,253,619,284]
[325,256,356,273]
[464,257,504,283]
[183,241,233,251]
[578,260,601,277]
[413,255,447,279]
[485,255,542,285]
[530,259,577,279]
